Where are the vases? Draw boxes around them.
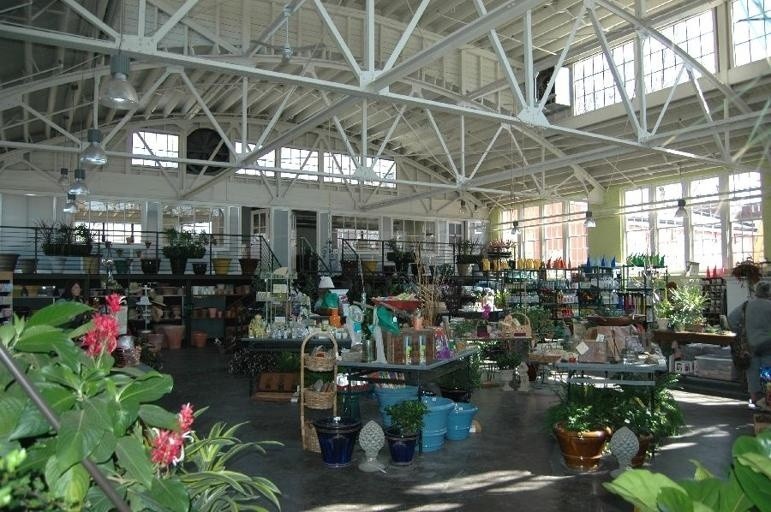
[1,253,265,352]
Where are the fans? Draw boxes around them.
[250,6,327,73]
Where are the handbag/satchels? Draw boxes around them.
[730,333,752,369]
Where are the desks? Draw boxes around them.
[238,337,352,399]
[655,329,738,382]
[556,362,659,418]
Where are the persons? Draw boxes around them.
[728,280,771,412]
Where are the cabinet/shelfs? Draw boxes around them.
[381,264,671,335]
[700,277,728,327]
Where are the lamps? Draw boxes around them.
[583,184,597,228]
[675,162,688,218]
[511,193,521,234]
[56,0,139,213]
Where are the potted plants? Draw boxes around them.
[386,236,419,262]
[33,217,98,256]
[542,369,690,470]
[161,226,218,258]
[450,236,517,264]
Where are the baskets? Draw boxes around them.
[305,420,322,454]
[304,385,336,410]
[502,312,531,337]
[303,352,337,372]
